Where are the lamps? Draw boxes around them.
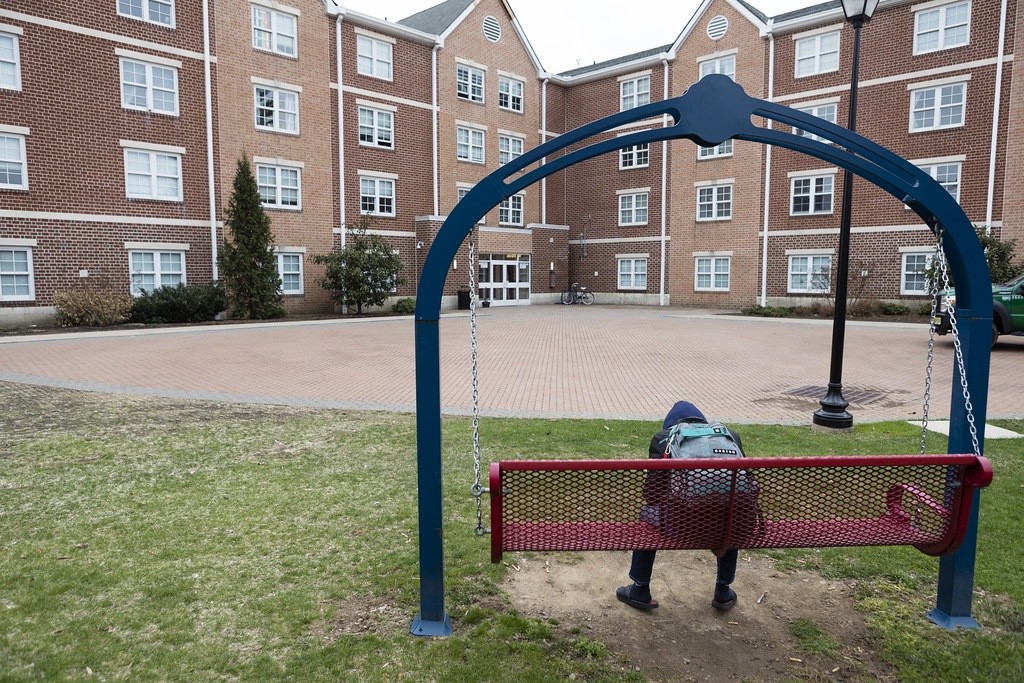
[416,241,424,249]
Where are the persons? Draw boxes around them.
[616,400,759,611]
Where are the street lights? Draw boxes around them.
[813,0,886,430]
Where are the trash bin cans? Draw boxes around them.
[457,290,473,309]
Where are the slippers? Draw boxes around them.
[616,583,659,610]
[712,588,737,611]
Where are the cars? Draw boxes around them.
[929,273,1024,348]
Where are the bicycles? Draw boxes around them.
[561,287,594,305]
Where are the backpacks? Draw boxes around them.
[665,421,751,514]
